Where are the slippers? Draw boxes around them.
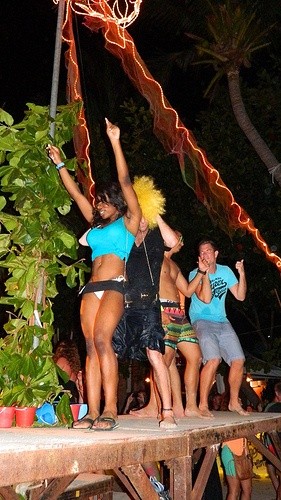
[90,414,120,431]
[72,415,98,429]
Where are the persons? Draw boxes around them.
[124,391,147,415]
[158,231,210,418]
[256,402,264,412]
[46,117,142,431]
[189,239,250,417]
[212,394,226,410]
[264,383,281,500]
[111,175,179,428]
[54,339,81,403]
[221,398,255,500]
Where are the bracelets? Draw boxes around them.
[56,163,65,170]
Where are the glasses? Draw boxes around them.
[178,235,185,243]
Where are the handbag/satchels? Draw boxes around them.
[232,453,255,480]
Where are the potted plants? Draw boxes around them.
[0,97,83,426]
